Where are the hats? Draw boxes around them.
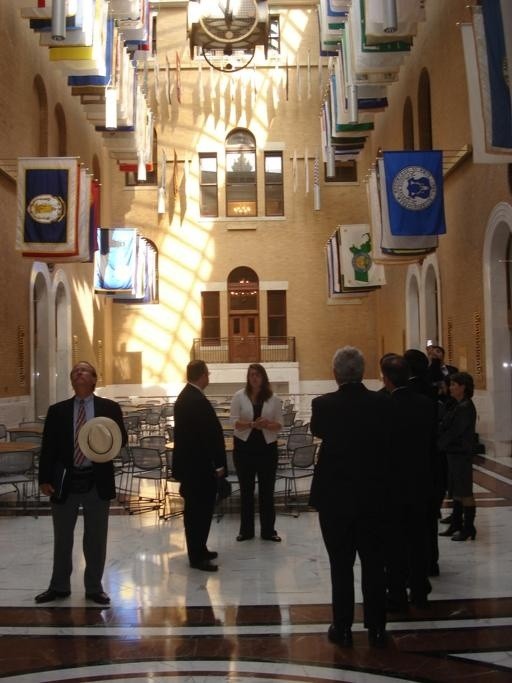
[78,417,122,463]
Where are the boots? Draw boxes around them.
[439,499,476,541]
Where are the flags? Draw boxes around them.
[183,157,190,196]
[315,0,447,307]
[16,0,157,305]
[159,150,167,197]
[458,0,512,164]
[173,151,178,197]
[155,49,313,105]
[292,152,299,193]
[304,149,310,196]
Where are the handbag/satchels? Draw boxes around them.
[50,468,70,504]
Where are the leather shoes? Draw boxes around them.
[85,592,110,603]
[35,590,70,602]
[236,534,281,542]
[328,624,389,646]
[191,552,218,571]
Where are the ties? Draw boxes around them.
[75,400,85,464]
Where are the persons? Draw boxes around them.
[310,343,479,651]
[35,361,128,606]
[227,363,282,543]
[172,358,230,571]
